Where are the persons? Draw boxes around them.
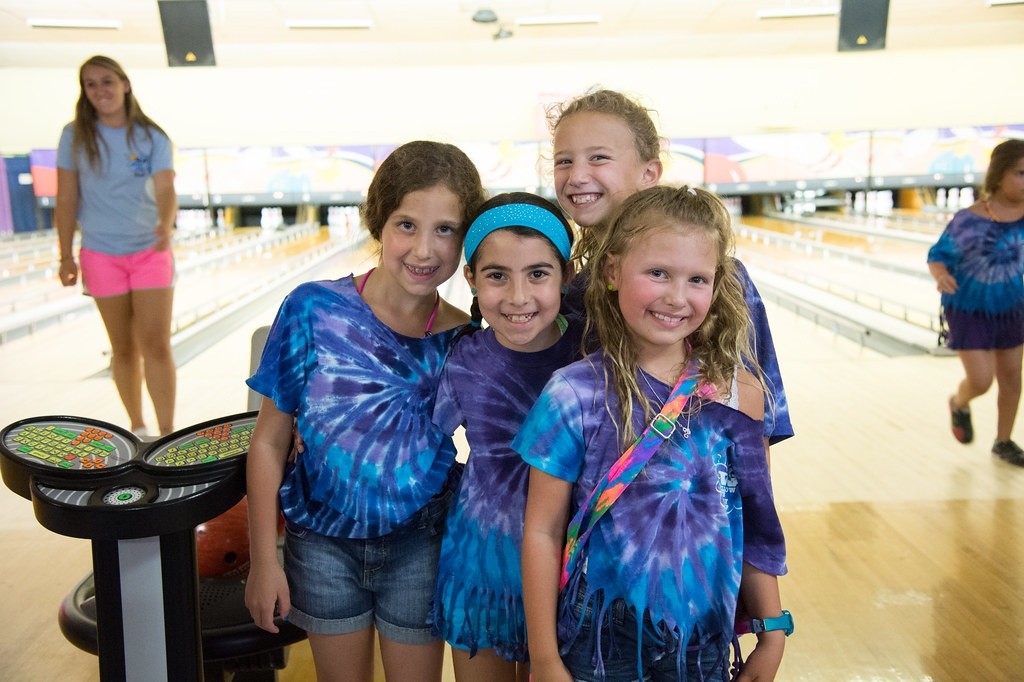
[241,90,796,682]
[926,139,1024,467]
[53,56,176,441]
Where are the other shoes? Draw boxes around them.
[992,439,1024,467]
[950,396,975,443]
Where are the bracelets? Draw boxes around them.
[59,256,74,262]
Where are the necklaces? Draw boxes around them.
[984,200,1000,222]
[357,267,441,336]
[636,357,693,438]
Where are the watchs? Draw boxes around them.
[753,611,794,637]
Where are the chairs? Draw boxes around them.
[58,323,310,682]
